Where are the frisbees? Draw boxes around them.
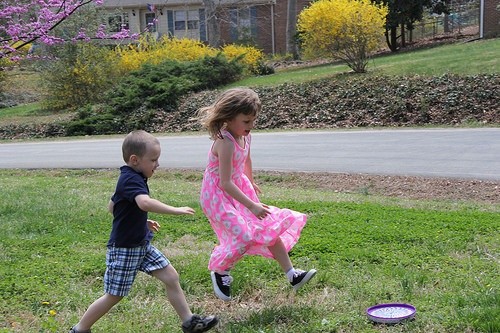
[366,302,417,324]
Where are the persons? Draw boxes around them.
[195,87,318,300]
[66,129,218,333]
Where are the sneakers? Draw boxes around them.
[289,269,316,291]
[211,271,231,301]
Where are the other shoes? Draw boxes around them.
[69,324,91,333]
[181,314,219,333]
